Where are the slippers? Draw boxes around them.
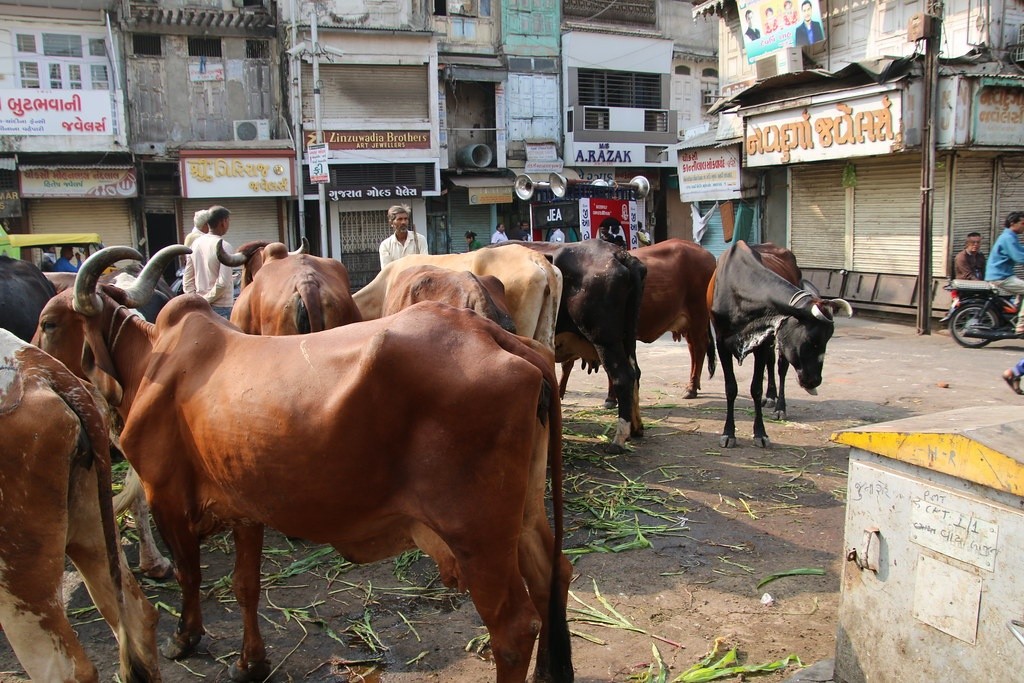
[1016,328,1024,335]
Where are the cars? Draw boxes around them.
[170,267,243,301]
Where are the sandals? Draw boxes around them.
[1002,369,1024,395]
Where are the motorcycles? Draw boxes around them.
[939,278,1024,348]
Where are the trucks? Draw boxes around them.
[0,224,119,295]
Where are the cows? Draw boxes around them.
[381,264,517,335]
[487,240,647,456]
[554,238,718,400]
[348,245,562,350]
[0,327,162,683]
[29,246,575,683]
[42,262,174,323]
[703,238,855,448]
[0,255,56,343]
[218,235,364,335]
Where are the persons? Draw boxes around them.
[379,204,428,270]
[599,225,625,246]
[464,230,483,252]
[745,10,760,40]
[985,211,1024,334]
[491,223,509,244]
[796,0,824,46]
[1001,357,1024,396]
[637,220,651,248]
[518,221,530,241]
[51,246,82,273]
[953,233,986,282]
[183,205,234,317]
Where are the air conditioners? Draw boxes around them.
[645,113,665,131]
[233,119,269,142]
[703,89,719,105]
[585,113,603,129]
[756,45,803,82]
[1015,23,1024,62]
